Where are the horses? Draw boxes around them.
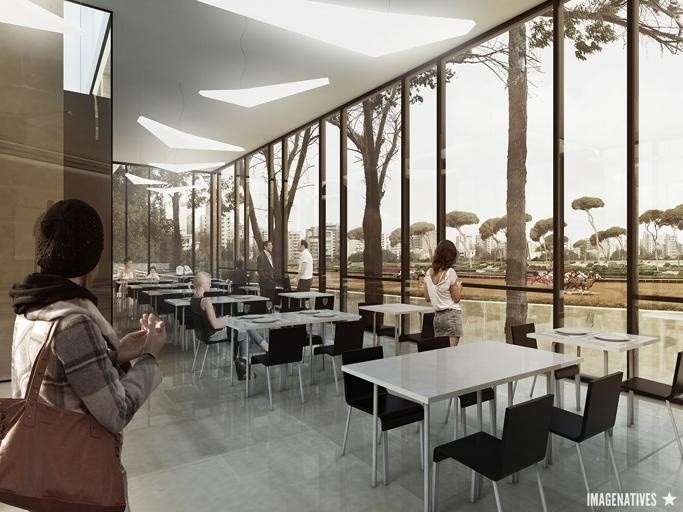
[531,272,602,297]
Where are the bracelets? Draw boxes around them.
[141,352,155,359]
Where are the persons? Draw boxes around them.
[9,199,167,511]
[226,259,248,284]
[296,239,314,290]
[421,240,463,346]
[189,271,269,379]
[120,257,137,279]
[257,241,277,305]
[146,266,160,280]
[175,257,191,276]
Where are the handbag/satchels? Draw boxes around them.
[0,316,128,512]
